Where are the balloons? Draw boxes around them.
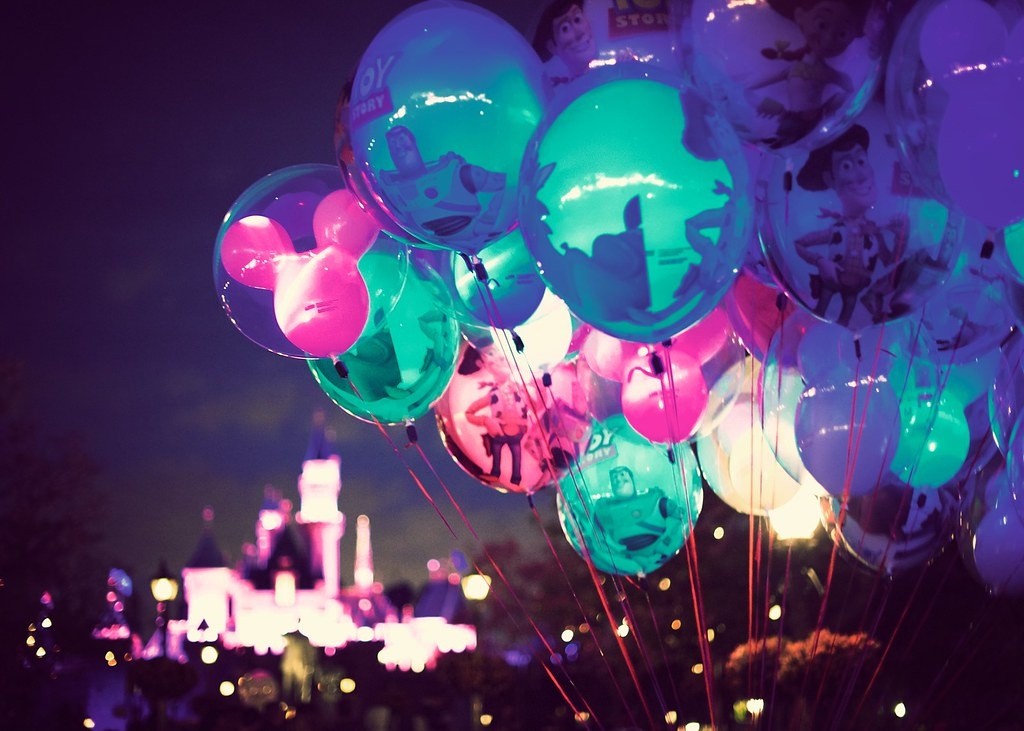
[299,254,462,430]
[329,0,1024,598]
[213,163,411,362]
